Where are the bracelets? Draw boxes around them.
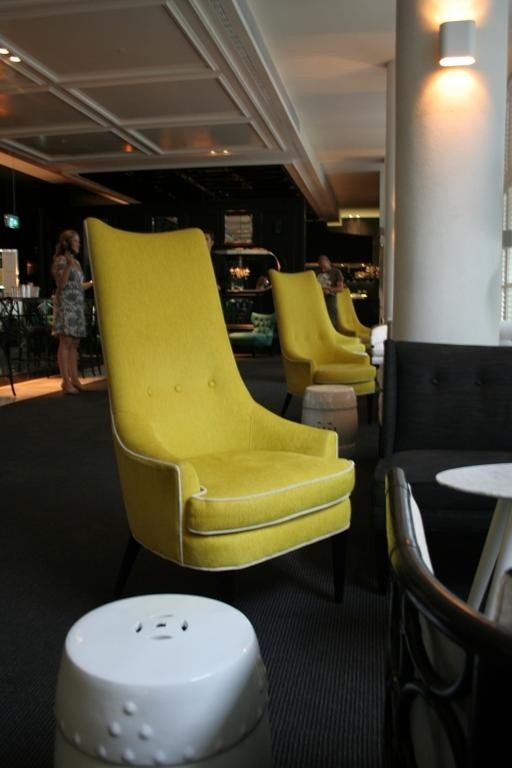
[90,280,93,286]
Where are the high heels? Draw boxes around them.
[61,381,87,395]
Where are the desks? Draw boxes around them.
[434,462,512,633]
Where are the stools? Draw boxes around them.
[50,591,273,767]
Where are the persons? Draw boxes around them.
[200,227,232,311]
[314,254,345,330]
[52,228,93,394]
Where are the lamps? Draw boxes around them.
[438,18,479,68]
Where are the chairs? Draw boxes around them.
[373,336,512,548]
[380,464,512,766]
[0,295,104,397]
